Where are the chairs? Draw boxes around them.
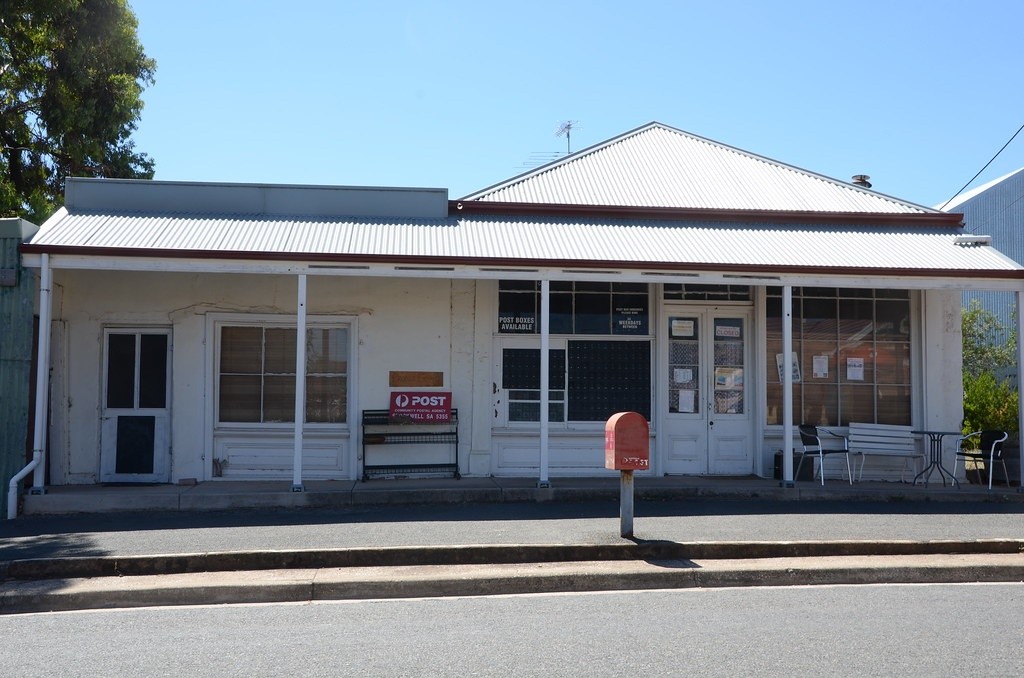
[952,429,1009,489]
[796,423,853,486]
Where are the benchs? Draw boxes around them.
[847,422,927,486]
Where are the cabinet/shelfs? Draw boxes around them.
[361,408,463,481]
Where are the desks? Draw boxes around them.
[908,429,966,489]
